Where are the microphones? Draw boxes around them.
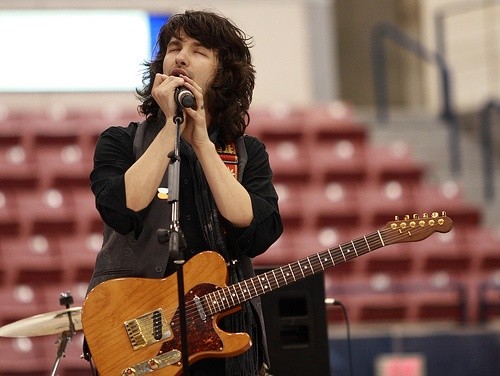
[175,86,195,108]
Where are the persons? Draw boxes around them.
[89,10,285,376]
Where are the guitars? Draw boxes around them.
[79,207,454,376]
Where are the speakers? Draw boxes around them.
[253,265,331,376]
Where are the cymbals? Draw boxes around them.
[0,305,84,338]
